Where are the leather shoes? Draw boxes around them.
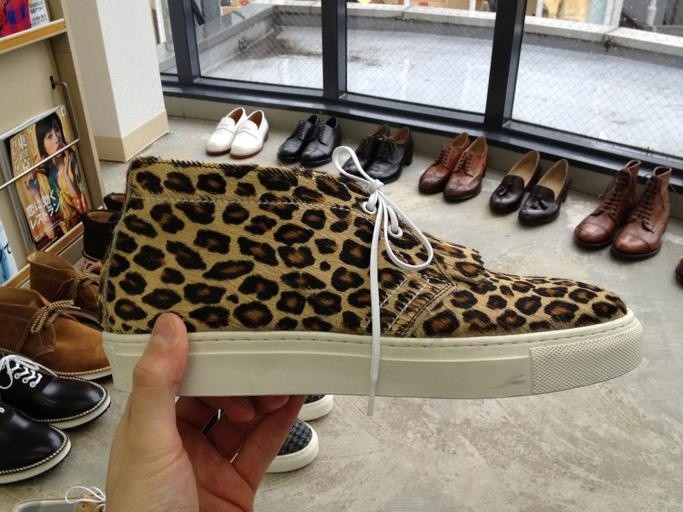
[490,150,572,225]
[418,132,489,202]
[342,124,413,184]
[0,355,111,484]
[206,106,270,157]
[278,115,342,168]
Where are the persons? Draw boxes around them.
[25,113,89,239]
[104,312,311,512]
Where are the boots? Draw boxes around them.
[0,286,113,381]
[575,159,672,259]
[27,252,102,315]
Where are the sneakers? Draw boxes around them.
[79,193,126,261]
[96,157,642,413]
[265,393,334,474]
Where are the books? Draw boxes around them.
[0,1,53,42]
[0,105,93,255]
